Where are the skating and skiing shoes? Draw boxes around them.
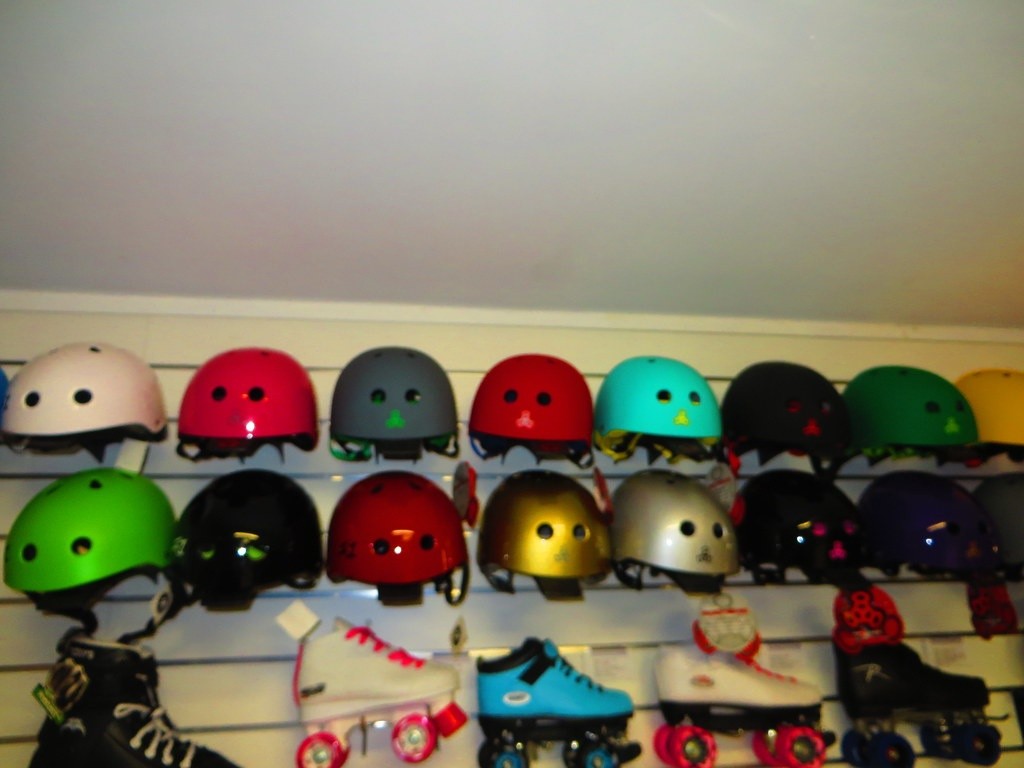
[292,617,468,768]
[657,639,836,768]
[477,636,635,767]
[842,635,1002,768]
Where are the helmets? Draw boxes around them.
[6,465,174,594]
[178,346,319,458]
[1,342,166,450]
[973,472,1024,570]
[728,467,857,575]
[173,468,321,591]
[858,471,995,568]
[719,360,854,465]
[469,353,594,447]
[956,369,1023,446]
[841,365,978,447]
[477,469,611,578]
[606,468,739,576]
[330,346,456,442]
[327,470,467,582]
[594,354,721,437]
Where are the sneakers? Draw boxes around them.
[27,627,243,768]
